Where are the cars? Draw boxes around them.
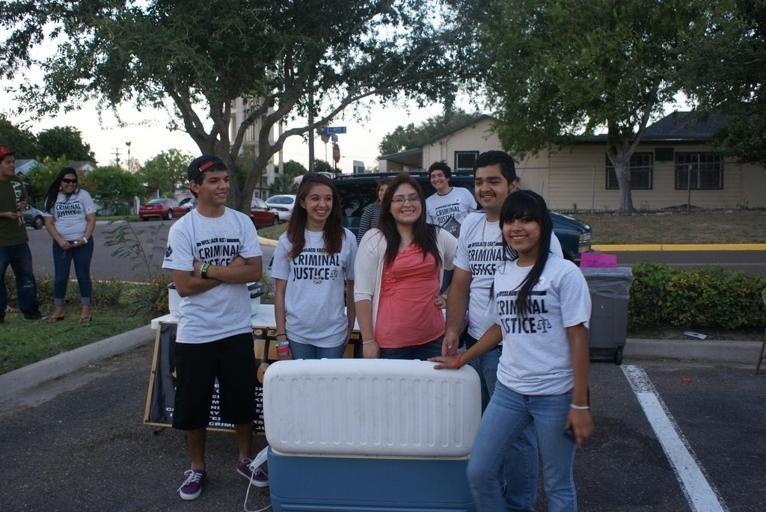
[137,192,296,231]
[19,203,47,229]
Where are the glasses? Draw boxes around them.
[62,178,76,183]
[392,196,419,205]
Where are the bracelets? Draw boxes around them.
[362,338,377,344]
[273,332,289,353]
[451,352,462,367]
[198,260,209,280]
[81,235,89,245]
[570,404,588,411]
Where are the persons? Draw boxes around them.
[353,175,460,360]
[429,191,595,512]
[425,159,478,236]
[159,155,269,503]
[0,146,43,320]
[43,167,98,323]
[267,170,359,359]
[439,149,522,403]
[356,180,391,247]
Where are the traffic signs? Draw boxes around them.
[331,135,339,142]
[324,127,345,134]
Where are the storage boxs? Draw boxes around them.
[168,281,263,317]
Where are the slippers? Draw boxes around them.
[46,315,64,323]
[80,314,92,323]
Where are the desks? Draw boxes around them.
[144,302,360,434]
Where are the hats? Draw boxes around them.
[0,146,14,159]
[187,155,222,180]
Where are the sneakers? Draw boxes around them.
[237,457,269,487]
[176,469,207,500]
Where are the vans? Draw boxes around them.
[289,172,593,266]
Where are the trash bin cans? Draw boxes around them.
[579,267,635,366]
